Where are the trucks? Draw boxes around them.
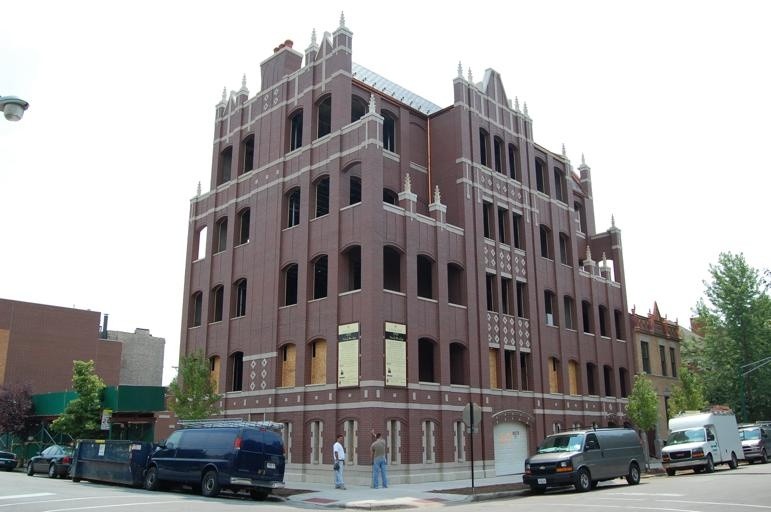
[662,406,746,475]
[738,420,771,464]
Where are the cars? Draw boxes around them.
[25,444,76,478]
[0,449,18,471]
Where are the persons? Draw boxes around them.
[370,433,388,489]
[333,435,348,491]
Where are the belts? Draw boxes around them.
[339,460,344,461]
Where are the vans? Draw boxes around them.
[523,428,646,493]
[143,427,286,504]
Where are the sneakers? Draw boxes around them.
[335,485,347,490]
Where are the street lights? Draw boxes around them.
[0,95,28,122]
